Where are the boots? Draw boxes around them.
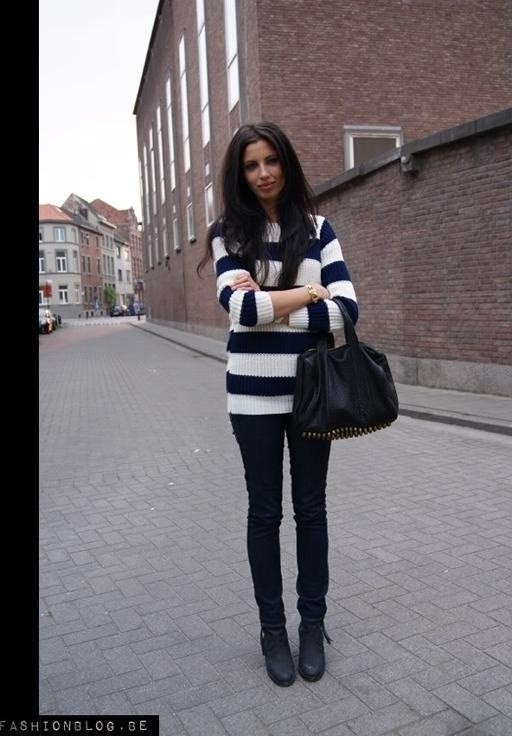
[260,617,296,688]
[297,614,327,684]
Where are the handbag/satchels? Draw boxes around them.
[292,297,399,442]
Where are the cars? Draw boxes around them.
[38,308,57,334]
[109,304,125,317]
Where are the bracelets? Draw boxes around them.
[305,285,319,303]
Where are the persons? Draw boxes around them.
[197,122,358,686]
